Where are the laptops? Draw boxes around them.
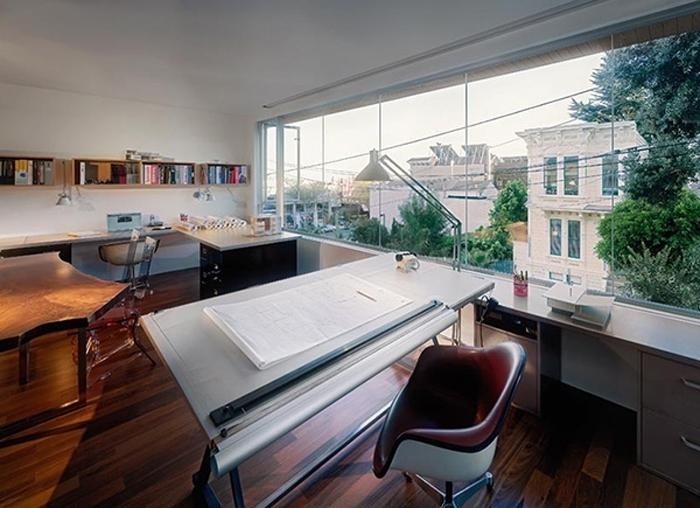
[107,212,152,239]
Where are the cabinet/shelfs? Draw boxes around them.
[0,152,250,191]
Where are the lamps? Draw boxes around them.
[353,148,463,346]
[55,186,72,206]
[191,187,217,202]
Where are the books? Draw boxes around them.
[77,160,248,185]
[202,272,415,370]
[1,157,54,188]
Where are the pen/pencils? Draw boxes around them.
[357,290,376,302]
[514,270,528,280]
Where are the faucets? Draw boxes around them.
[565,267,573,288]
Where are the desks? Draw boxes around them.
[139,252,495,508]
[0,250,131,398]
[468,276,700,498]
[1,216,302,299]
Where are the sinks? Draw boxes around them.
[543,281,586,314]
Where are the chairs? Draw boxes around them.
[372,342,528,508]
[98,238,161,298]
